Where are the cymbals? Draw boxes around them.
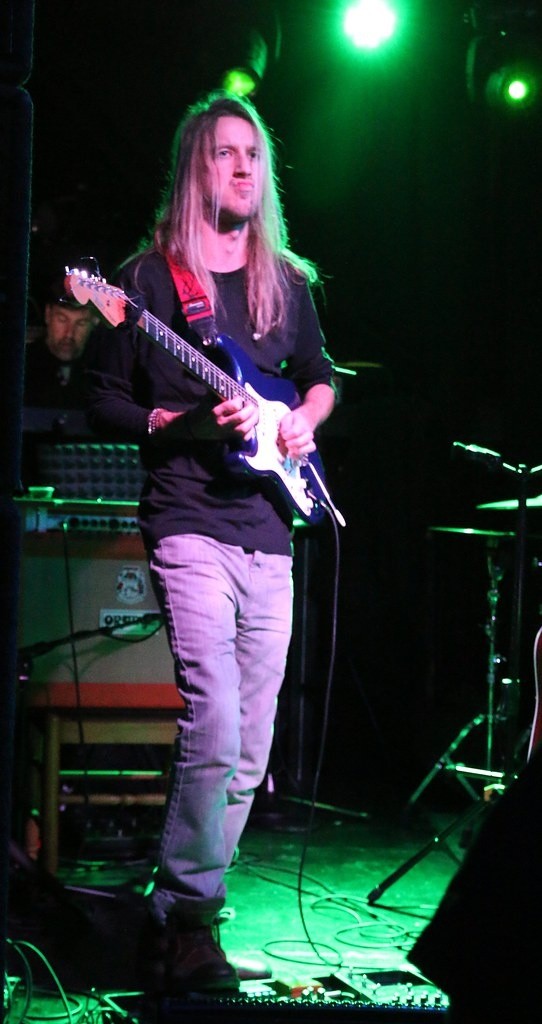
[476,492,542,510]
[428,525,517,537]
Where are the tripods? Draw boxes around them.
[402,536,529,822]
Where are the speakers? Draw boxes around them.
[14,494,190,710]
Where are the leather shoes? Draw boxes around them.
[143,902,240,991]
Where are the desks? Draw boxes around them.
[30,711,177,876]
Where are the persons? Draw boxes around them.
[23,256,108,409]
[83,88,339,996]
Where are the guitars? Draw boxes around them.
[61,261,330,530]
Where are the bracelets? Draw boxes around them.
[148,408,166,434]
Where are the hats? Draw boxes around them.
[44,256,101,307]
